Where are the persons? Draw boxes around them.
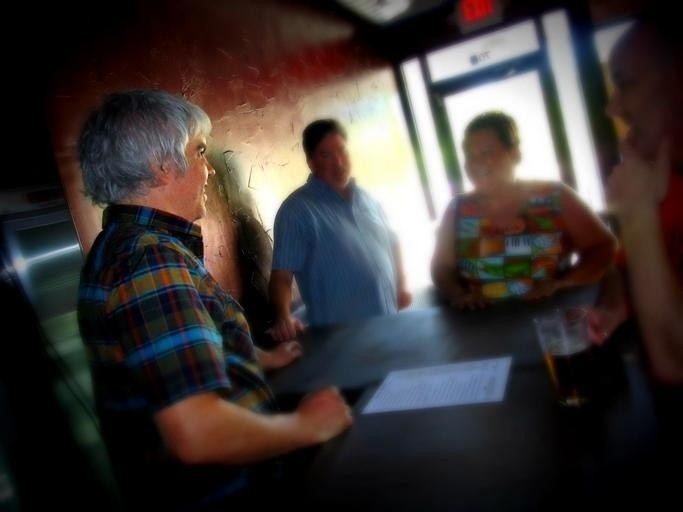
[606,11,682,391]
[268,119,411,342]
[431,109,621,312]
[73,88,354,512]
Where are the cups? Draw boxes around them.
[532,306,603,409]
